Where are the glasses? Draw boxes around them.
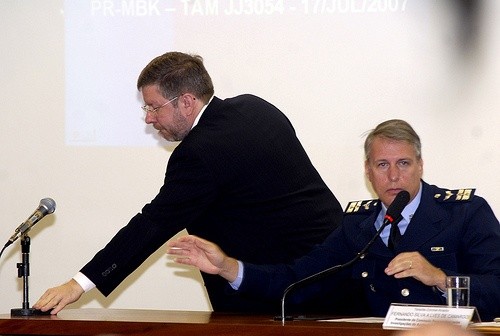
[141,94,195,119]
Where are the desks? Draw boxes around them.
[0,308,500,336]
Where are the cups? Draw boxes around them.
[445,276,470,308]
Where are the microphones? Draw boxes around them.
[5,198,56,248]
[281,190,410,326]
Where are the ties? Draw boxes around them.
[388,214,403,253]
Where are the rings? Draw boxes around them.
[409,263,411,269]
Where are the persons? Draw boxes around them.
[34,51,345,314]
[168,120,500,320]
